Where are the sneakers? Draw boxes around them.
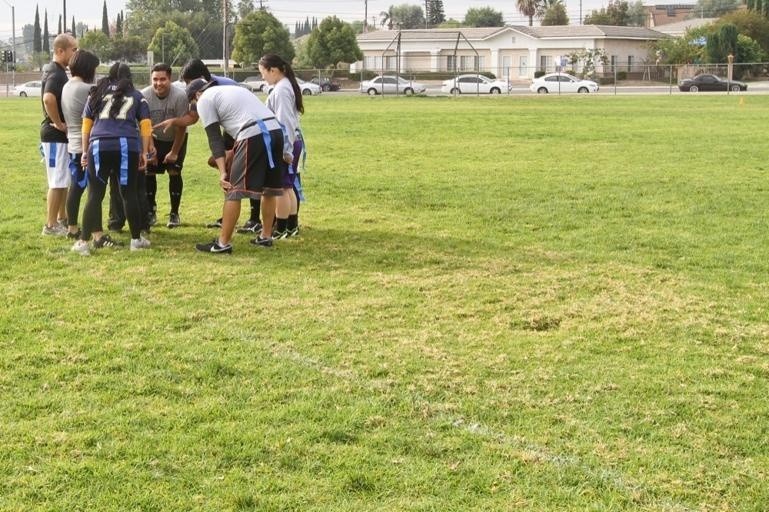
[42,212,299,258]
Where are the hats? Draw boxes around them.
[186,79,218,103]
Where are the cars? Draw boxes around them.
[529,72,601,95]
[357,74,427,96]
[14,80,43,96]
[309,76,341,91]
[238,76,267,92]
[262,75,323,95]
[439,71,514,95]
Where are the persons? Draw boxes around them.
[179,54,308,255]
[40,34,189,256]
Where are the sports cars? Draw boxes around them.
[676,73,747,92]
